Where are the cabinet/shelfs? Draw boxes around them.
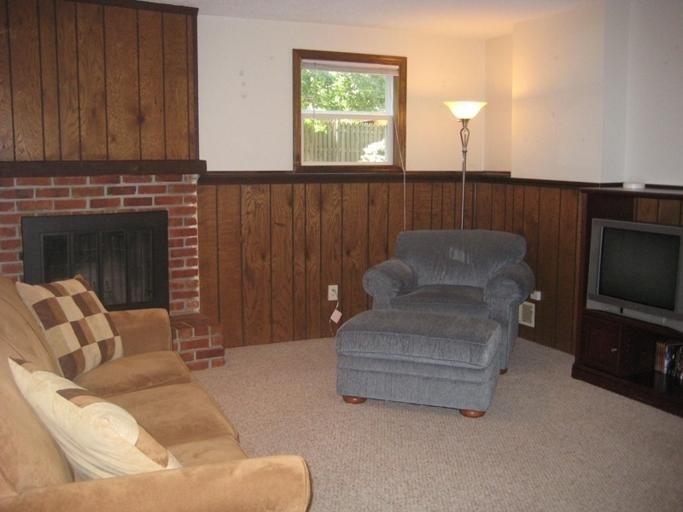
[570,311,682,418]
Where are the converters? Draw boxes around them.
[330,309,342,324]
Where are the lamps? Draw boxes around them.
[441,98,488,231]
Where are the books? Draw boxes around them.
[652,335,683,383]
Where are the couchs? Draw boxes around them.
[1,275,314,511]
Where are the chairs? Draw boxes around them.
[336,229,537,418]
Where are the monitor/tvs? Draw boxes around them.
[586,217,683,333]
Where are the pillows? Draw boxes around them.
[4,270,186,482]
